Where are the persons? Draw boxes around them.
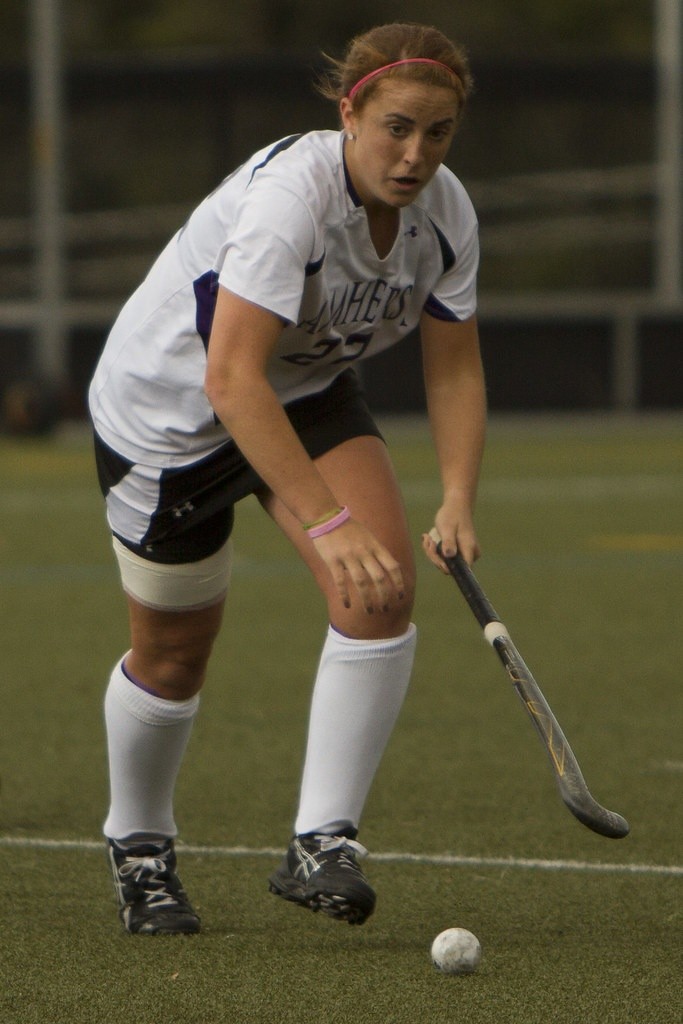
[88,25,486,934]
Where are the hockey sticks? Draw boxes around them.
[428,525,632,840]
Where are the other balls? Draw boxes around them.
[432,927,481,976]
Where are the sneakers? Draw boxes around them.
[107,835,200,935]
[268,820,376,926]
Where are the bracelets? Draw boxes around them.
[304,505,351,538]
